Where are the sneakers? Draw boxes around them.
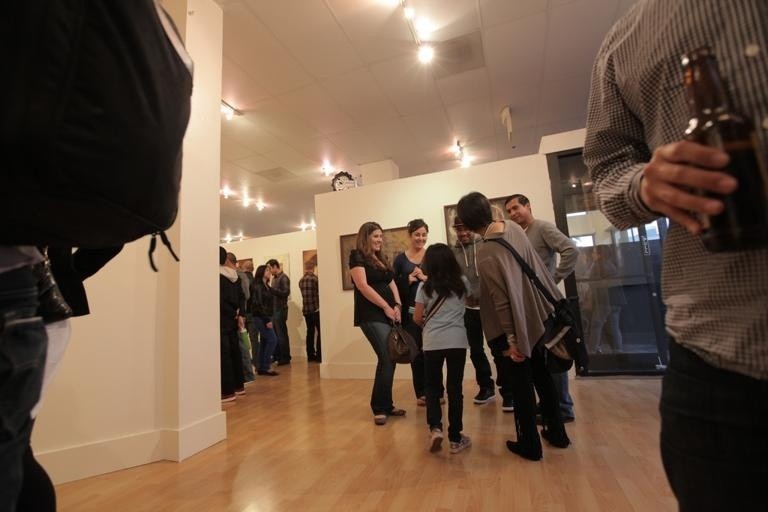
[221,390,246,402]
[389,407,406,416]
[507,440,540,461]
[428,428,444,454]
[501,398,514,411]
[450,433,471,454]
[374,413,386,424]
[473,391,495,403]
[243,360,290,385]
[536,402,575,425]
[541,429,568,448]
[417,396,445,406]
[308,354,321,362]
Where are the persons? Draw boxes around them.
[219,245,291,403]
[0,0,194,512]
[581,0,767,512]
[352,189,581,461]
[298,261,322,363]
[589,238,626,353]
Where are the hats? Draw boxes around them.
[452,216,464,227]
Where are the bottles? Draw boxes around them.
[680,47,768,253]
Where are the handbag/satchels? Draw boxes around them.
[532,298,582,378]
[387,323,423,363]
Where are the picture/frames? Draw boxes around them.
[339,226,411,292]
[444,195,515,248]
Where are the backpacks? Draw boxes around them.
[0,0,192,245]
